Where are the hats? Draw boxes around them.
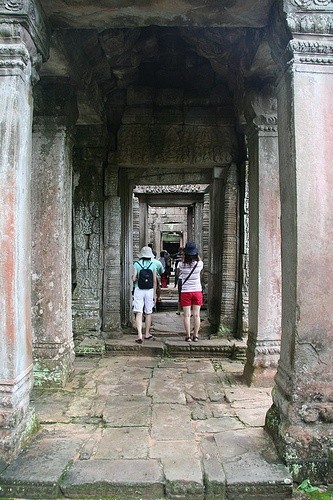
[185,242,197,255]
[136,246,155,259]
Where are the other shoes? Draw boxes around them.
[175,311,180,315]
[135,339,143,344]
[144,334,153,339]
[185,337,192,342]
[193,337,199,342]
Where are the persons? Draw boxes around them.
[132,246,156,340]
[180,242,203,342]
[144,244,192,316]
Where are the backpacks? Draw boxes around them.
[137,261,153,289]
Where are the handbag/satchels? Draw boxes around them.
[178,279,182,293]
